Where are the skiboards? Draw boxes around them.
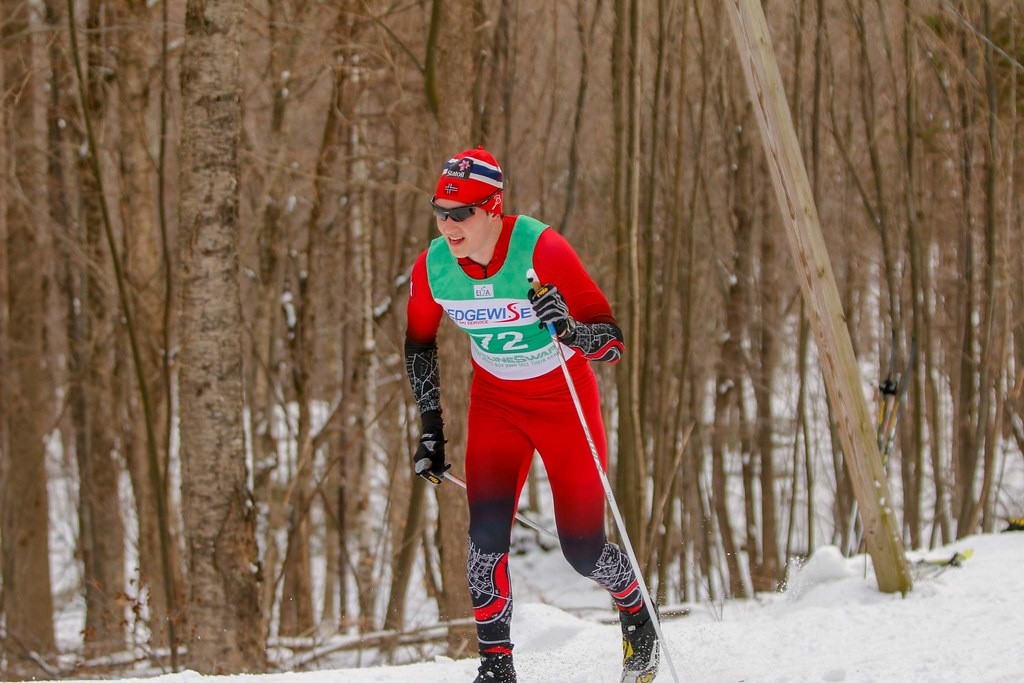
[618,604,663,682]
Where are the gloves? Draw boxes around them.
[413,409,451,485]
[527,283,570,335]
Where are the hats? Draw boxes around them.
[432,145,504,215]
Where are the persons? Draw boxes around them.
[405,145,661,683]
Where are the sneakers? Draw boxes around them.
[618,596,661,683]
[472,650,517,683]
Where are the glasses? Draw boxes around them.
[429,188,503,222]
